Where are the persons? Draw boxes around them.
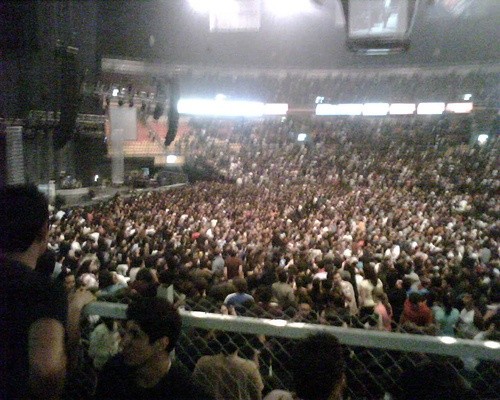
[33,69,500,400]
[0,181,68,399]
